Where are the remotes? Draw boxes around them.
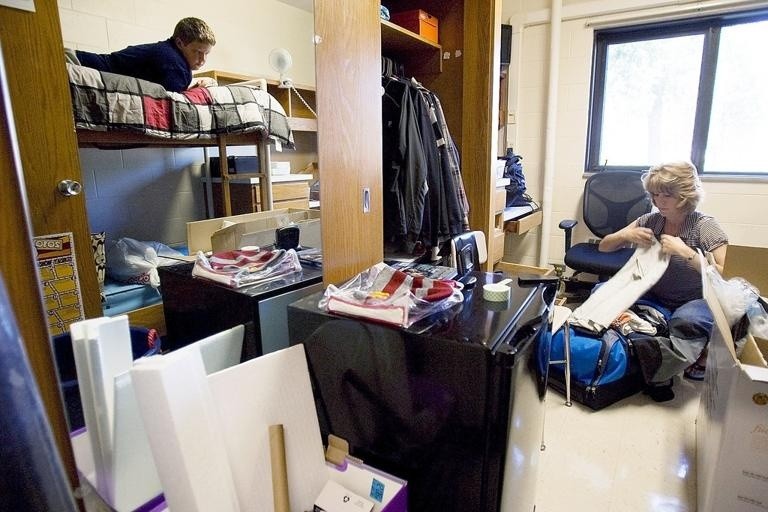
[517,274,561,286]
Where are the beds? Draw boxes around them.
[65,78,268,339]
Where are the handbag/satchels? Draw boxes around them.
[534,300,670,411]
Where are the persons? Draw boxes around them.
[63,17,218,96]
[596,157,729,381]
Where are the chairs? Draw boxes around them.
[558,171,653,283]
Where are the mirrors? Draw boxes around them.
[56,0,324,320]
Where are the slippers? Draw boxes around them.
[685,362,706,380]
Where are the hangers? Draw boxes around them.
[381,56,411,81]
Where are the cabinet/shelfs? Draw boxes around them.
[193,70,320,211]
[313,0,502,289]
[493,188,555,276]
[200,172,313,220]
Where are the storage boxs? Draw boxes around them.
[695,245,768,512]
[389,9,439,44]
[69,427,114,512]
[186,208,323,256]
[130,448,410,512]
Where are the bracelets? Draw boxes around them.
[685,251,696,263]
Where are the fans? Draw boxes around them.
[269,47,294,90]
